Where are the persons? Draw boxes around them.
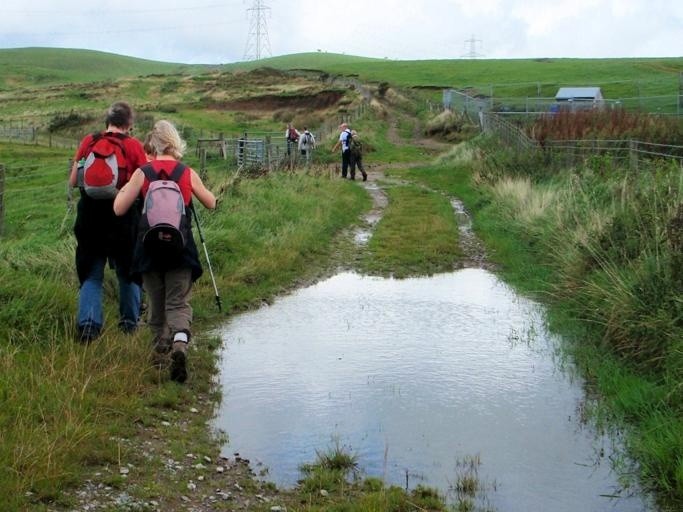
[332,123,368,181]
[285,123,315,156]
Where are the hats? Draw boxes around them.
[108,101,133,118]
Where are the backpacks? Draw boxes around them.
[344,131,363,154]
[84,131,130,199]
[303,132,313,146]
[289,128,298,140]
[137,163,190,250]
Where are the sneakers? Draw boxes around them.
[341,173,367,182]
[120,325,136,335]
[149,336,187,382]
[78,325,100,341]
